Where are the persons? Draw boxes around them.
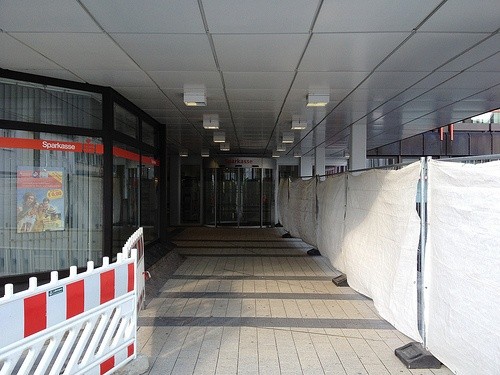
[18,192,58,231]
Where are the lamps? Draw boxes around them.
[306,94,330,107]
[183,93,208,108]
[290,120,307,130]
[202,119,220,130]
[272,135,295,158]
[179,136,231,159]
